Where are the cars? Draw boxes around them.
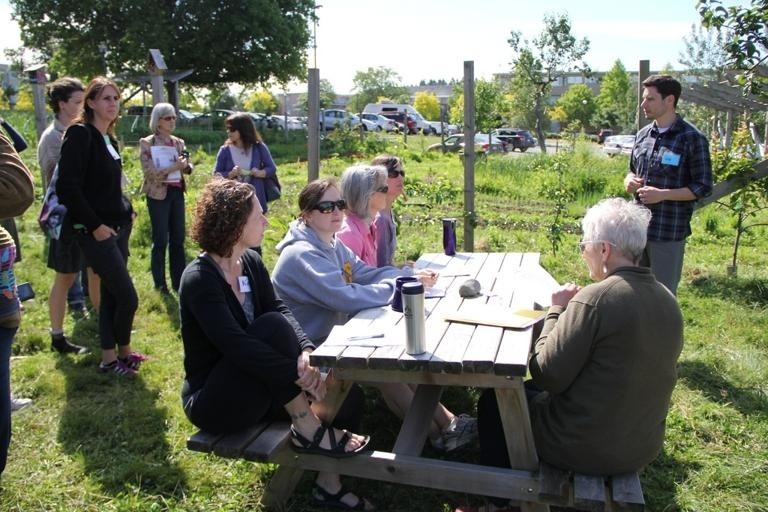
[597,126,622,145]
[427,129,539,158]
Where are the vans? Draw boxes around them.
[603,134,636,158]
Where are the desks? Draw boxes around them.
[259,249,552,511]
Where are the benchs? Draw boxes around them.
[539,458,646,510]
[187,341,355,511]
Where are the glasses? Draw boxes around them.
[388,170,404,178]
[227,127,236,132]
[160,116,176,120]
[313,200,345,213]
[377,185,388,192]
[580,239,616,251]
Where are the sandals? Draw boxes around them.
[291,421,371,458]
[311,484,374,511]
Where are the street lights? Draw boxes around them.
[582,99,587,113]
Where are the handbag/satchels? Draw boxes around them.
[39,163,67,240]
[263,174,280,202]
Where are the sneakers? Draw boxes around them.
[442,416,479,452]
[100,351,146,375]
[11,398,32,409]
[52,336,90,353]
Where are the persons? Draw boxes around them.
[139,103,193,295]
[175,174,379,512]
[456,194,685,510]
[56,80,150,380]
[0,132,32,411]
[370,153,416,268]
[0,229,21,472]
[39,78,101,353]
[336,162,389,267]
[623,74,714,297]
[213,113,276,258]
[269,178,479,453]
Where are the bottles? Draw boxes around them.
[399,279,427,357]
[179,150,189,160]
[441,218,457,256]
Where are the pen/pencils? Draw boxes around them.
[346,334,383,340]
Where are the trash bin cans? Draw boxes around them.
[597,129,613,144]
